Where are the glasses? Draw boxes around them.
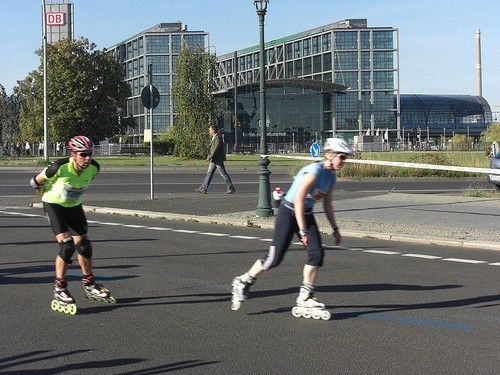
[75,152,93,157]
[336,155,347,160]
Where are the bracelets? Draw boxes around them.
[333,227,339,230]
[298,228,309,237]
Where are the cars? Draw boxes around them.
[488,151,500,188]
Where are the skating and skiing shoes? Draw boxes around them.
[81,273,116,303]
[52,279,76,314]
[292,285,330,320]
[231,273,256,310]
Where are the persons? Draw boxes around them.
[30,136,110,303]
[4,138,10,157]
[56,139,66,157]
[38,140,44,156]
[195,125,236,195]
[16,140,31,156]
[237,139,353,310]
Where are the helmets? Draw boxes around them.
[68,136,94,154]
[324,138,353,154]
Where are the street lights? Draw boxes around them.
[253,0,271,217]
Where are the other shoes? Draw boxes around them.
[224,188,236,194]
[195,188,207,194]
